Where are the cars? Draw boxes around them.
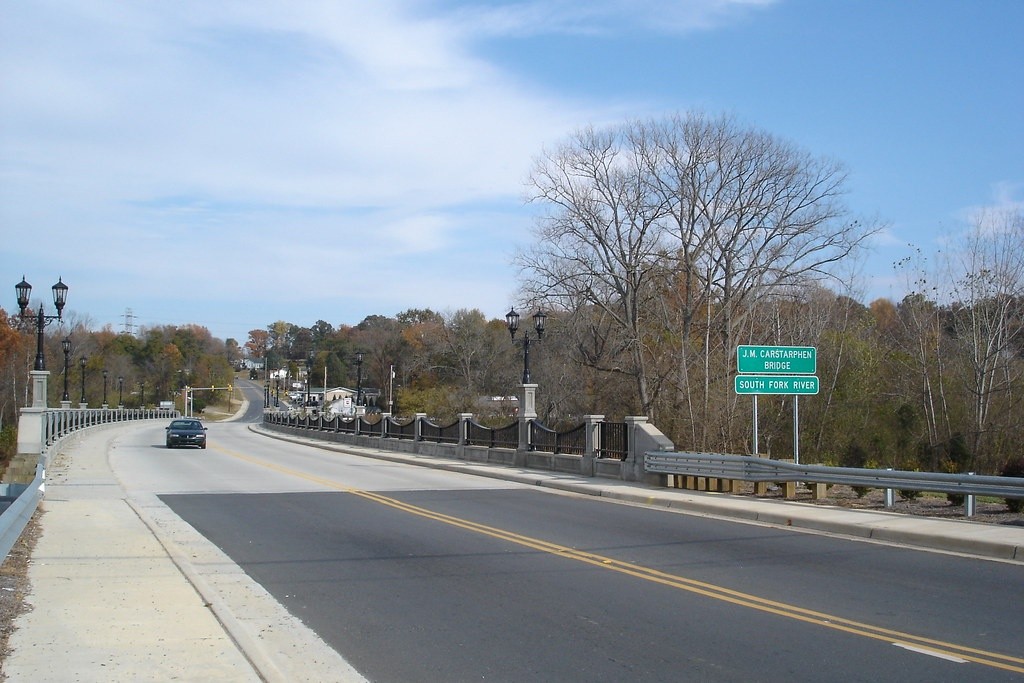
[165,420,208,449]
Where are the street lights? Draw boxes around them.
[156,386,159,406]
[15,273,69,372]
[141,382,144,406]
[118,376,124,406]
[102,369,108,404]
[80,356,87,403]
[274,375,280,407]
[356,349,364,407]
[505,304,547,384]
[264,380,271,408]
[306,348,314,407]
[62,336,71,401]
